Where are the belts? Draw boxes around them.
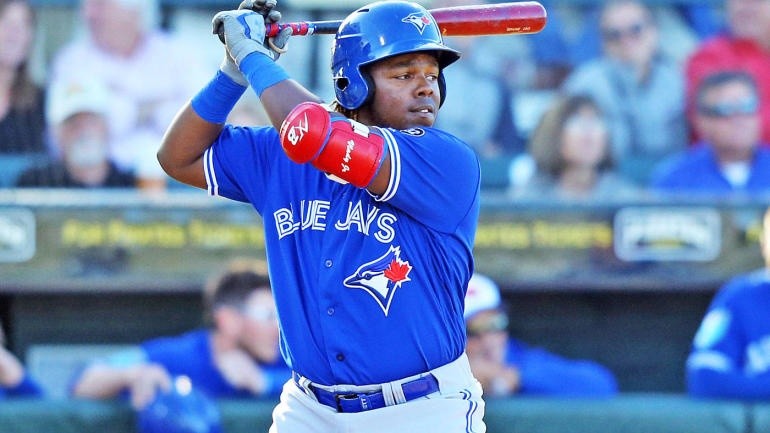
[292,371,439,413]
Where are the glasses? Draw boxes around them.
[464,313,509,338]
[697,100,758,118]
[602,20,645,42]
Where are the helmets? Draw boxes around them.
[331,1,462,110]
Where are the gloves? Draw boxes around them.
[211,9,273,71]
[225,0,293,71]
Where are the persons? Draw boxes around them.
[156,0,486,433]
[412,0,770,407]
[0,0,291,409]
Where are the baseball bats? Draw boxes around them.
[218,0,549,43]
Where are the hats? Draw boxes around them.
[464,274,501,317]
